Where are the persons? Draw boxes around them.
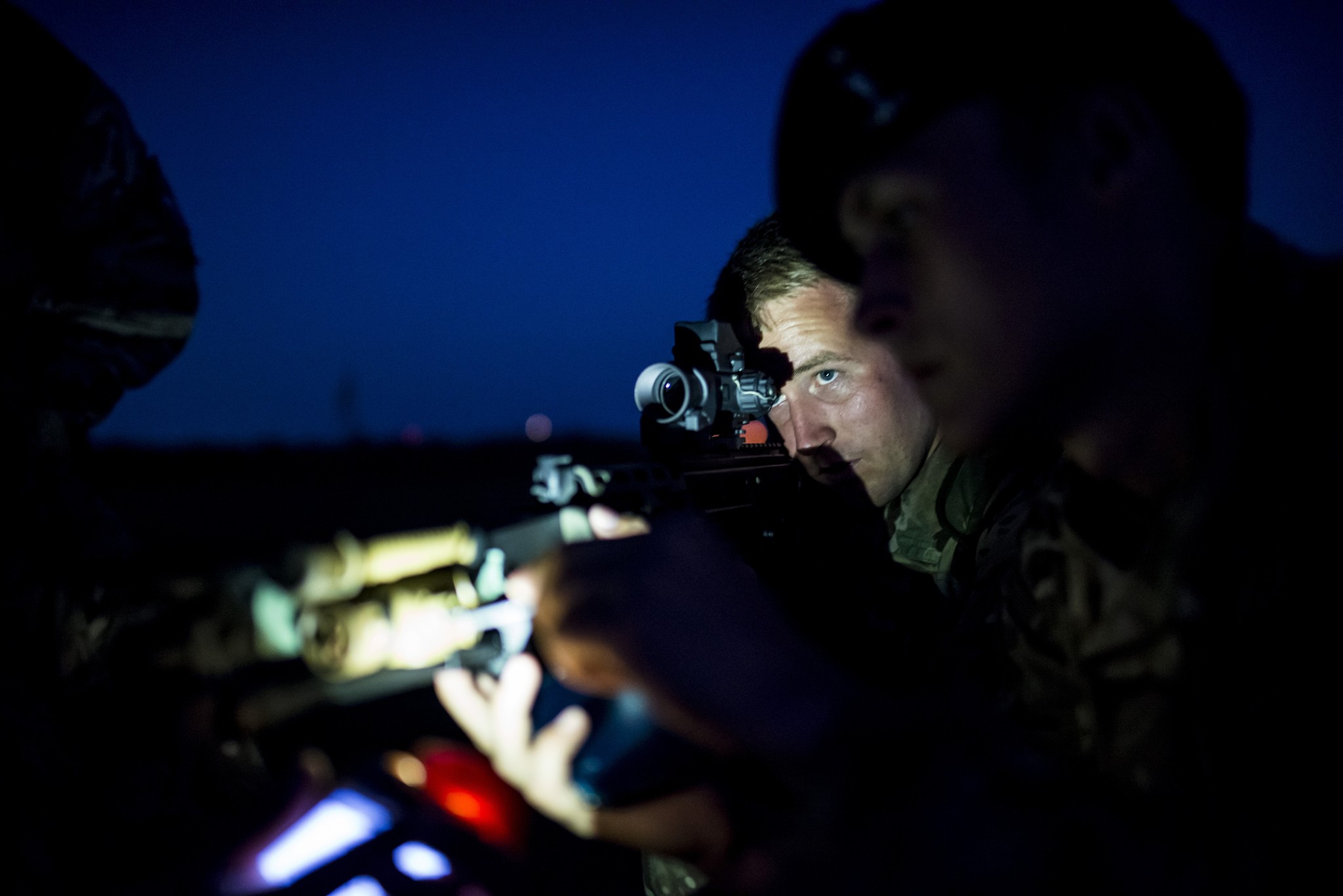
[430,2,1343,896]
[535,214,982,764]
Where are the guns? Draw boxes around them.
[533,317,814,546]
